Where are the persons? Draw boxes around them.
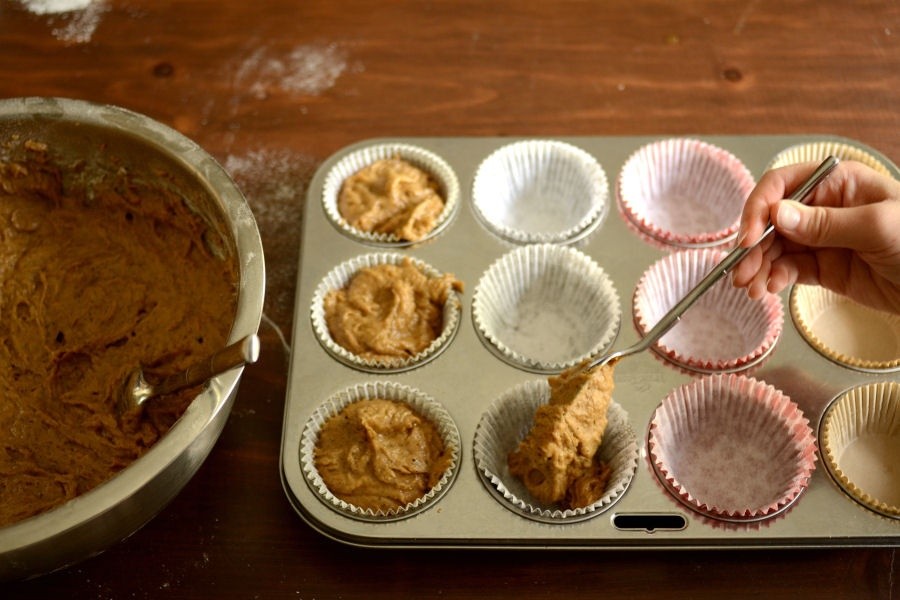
[732,160,900,315]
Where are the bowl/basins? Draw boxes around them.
[0,96,268,585]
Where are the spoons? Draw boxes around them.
[114,334,260,416]
[535,152,840,408]
